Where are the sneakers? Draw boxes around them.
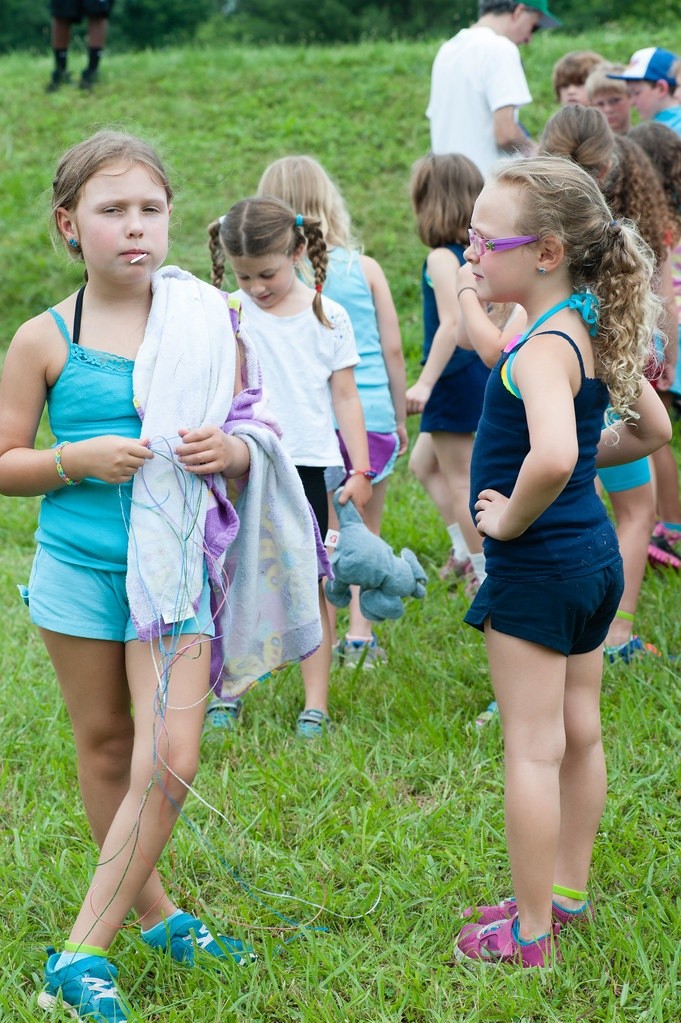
[463,577,482,600]
[36,945,143,1023]
[204,695,244,730]
[646,523,681,573]
[436,549,474,581]
[294,708,333,742]
[139,907,260,979]
[344,630,379,672]
[453,908,564,979]
[468,700,499,733]
[602,633,677,667]
[458,889,596,945]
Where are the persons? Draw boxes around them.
[45,0,114,91]
[0,131,252,1023]
[254,155,409,670]
[407,47,681,733]
[454,159,667,971]
[426,0,560,183]
[207,194,374,737]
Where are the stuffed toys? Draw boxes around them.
[324,486,429,622]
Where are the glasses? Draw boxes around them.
[465,227,540,257]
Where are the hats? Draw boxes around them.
[477,0,564,31]
[604,46,681,90]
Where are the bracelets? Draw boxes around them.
[457,287,477,297]
[349,470,377,479]
[55,441,83,486]
